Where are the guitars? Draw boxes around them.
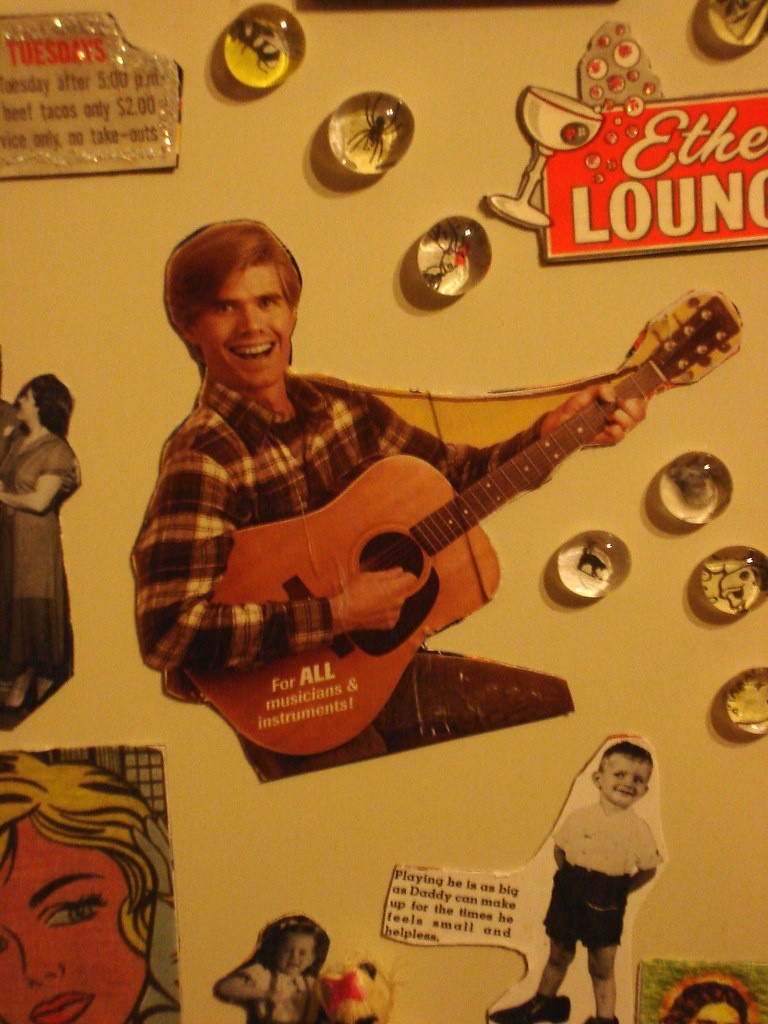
[168,284,746,760]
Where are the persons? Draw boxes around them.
[128,219,649,786]
[487,739,662,1024]
[0,372,84,728]
[212,915,331,1024]
